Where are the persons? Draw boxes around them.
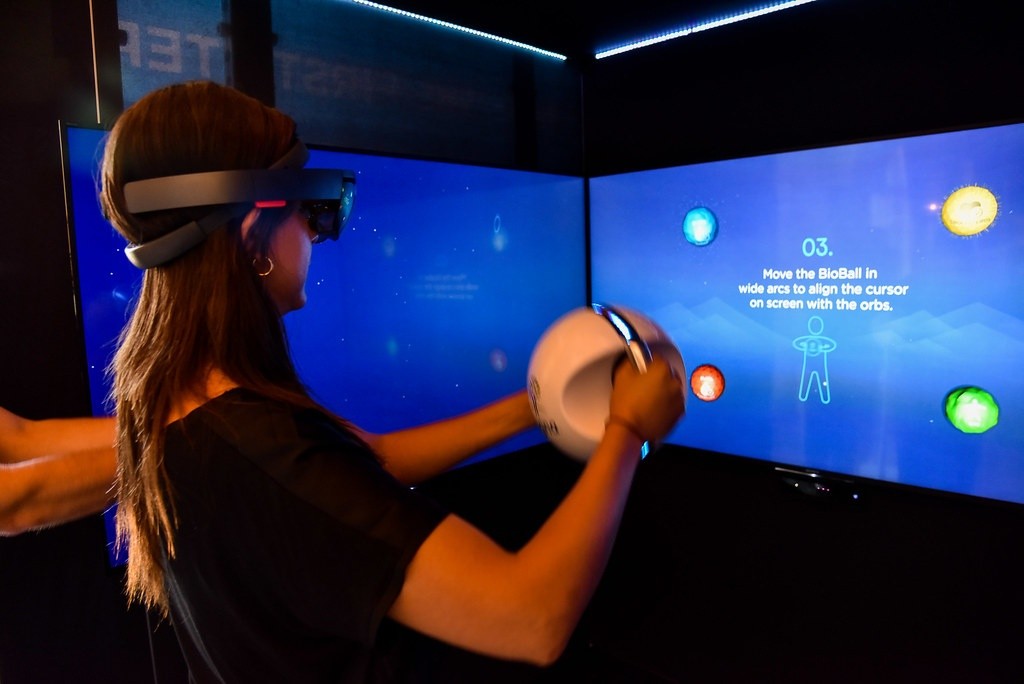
[0,406,120,536]
[100,78,687,684]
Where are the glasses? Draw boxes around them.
[297,172,355,246]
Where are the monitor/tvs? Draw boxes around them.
[53,119,1024,571]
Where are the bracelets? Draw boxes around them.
[604,416,644,444]
